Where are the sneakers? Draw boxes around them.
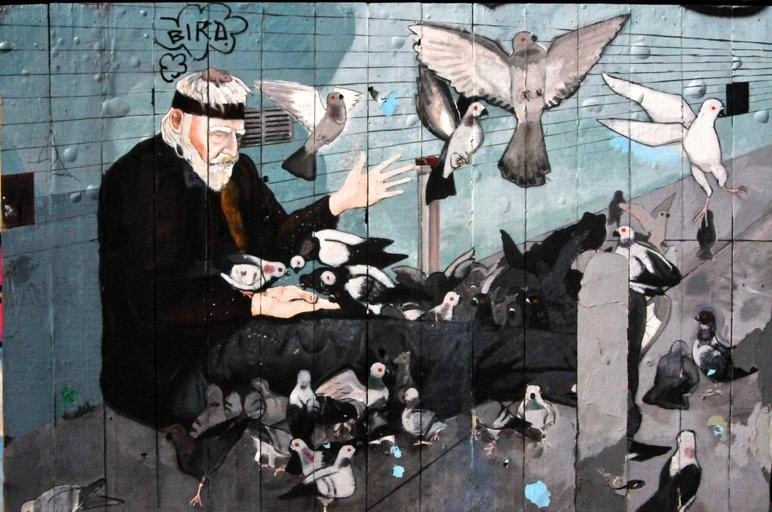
[630,290,670,438]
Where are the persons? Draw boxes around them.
[94,67,674,440]
